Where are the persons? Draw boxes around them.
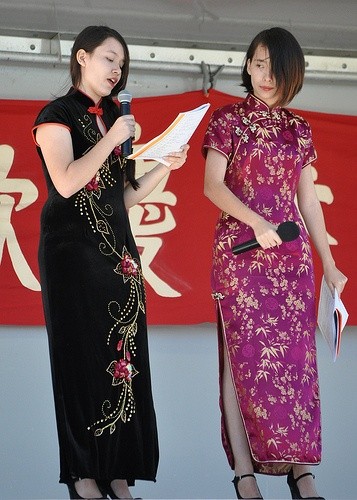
[201,27,348,500]
[29,24,192,500]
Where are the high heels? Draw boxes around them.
[232,474,264,500]
[104,479,143,500]
[287,466,325,500]
[67,476,109,500]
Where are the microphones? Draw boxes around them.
[232,221,299,254]
[118,90,132,156]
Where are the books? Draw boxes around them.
[126,101,212,168]
[317,274,350,367]
